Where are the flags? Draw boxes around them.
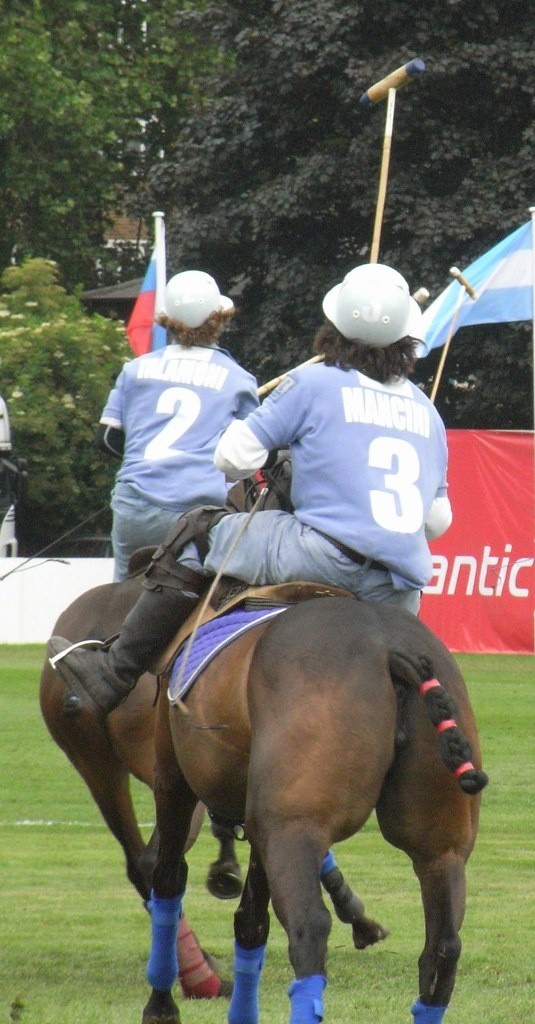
[413,217,534,358]
[125,212,167,357]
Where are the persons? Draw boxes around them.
[48,263,452,717]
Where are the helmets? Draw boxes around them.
[162,271,233,329]
[322,262,425,350]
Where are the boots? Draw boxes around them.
[45,577,201,725]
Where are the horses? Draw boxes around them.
[37,447,492,1024]
